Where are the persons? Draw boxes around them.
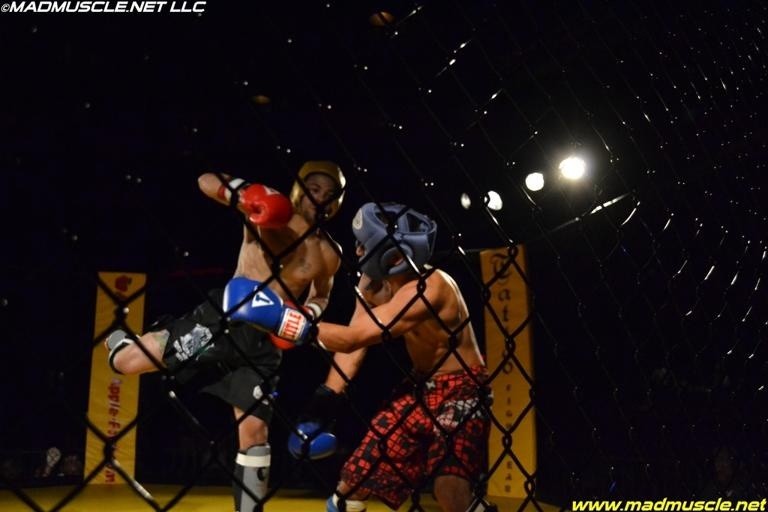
[101,158,349,512]
[221,198,500,511]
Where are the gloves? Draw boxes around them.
[222,275,322,350]
[287,384,339,465]
[217,174,294,228]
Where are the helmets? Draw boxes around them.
[289,160,349,226]
[352,201,438,284]
